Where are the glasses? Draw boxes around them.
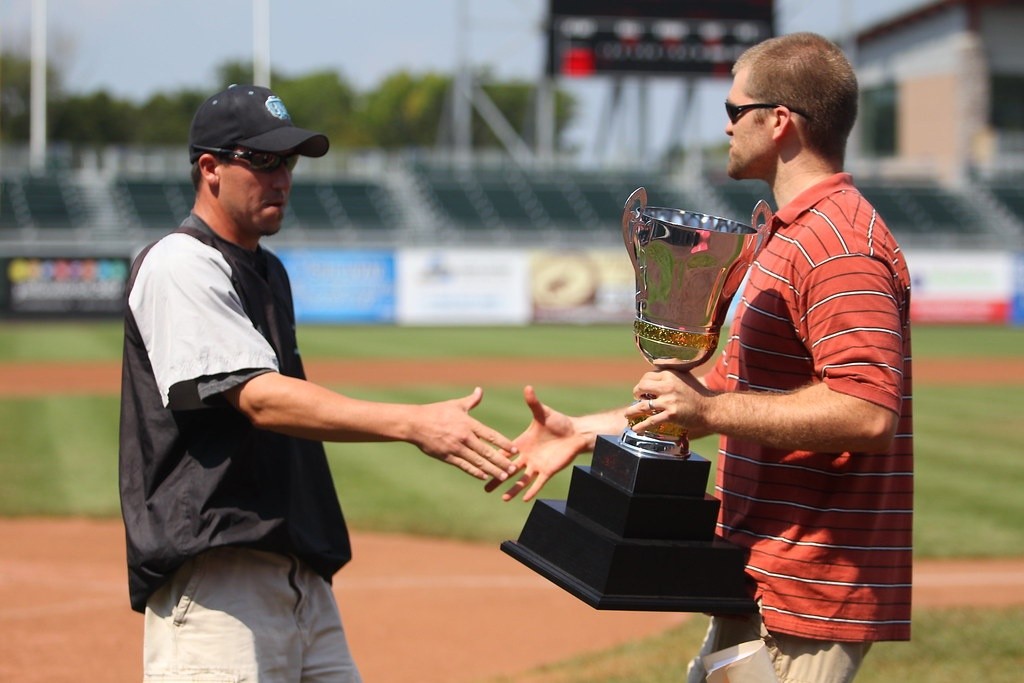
[725,100,812,124]
[193,143,300,171]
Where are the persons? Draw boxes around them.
[115,83,521,683]
[480,32,910,681]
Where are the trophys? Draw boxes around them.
[496,183,774,620]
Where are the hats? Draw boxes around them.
[188,85,328,162]
